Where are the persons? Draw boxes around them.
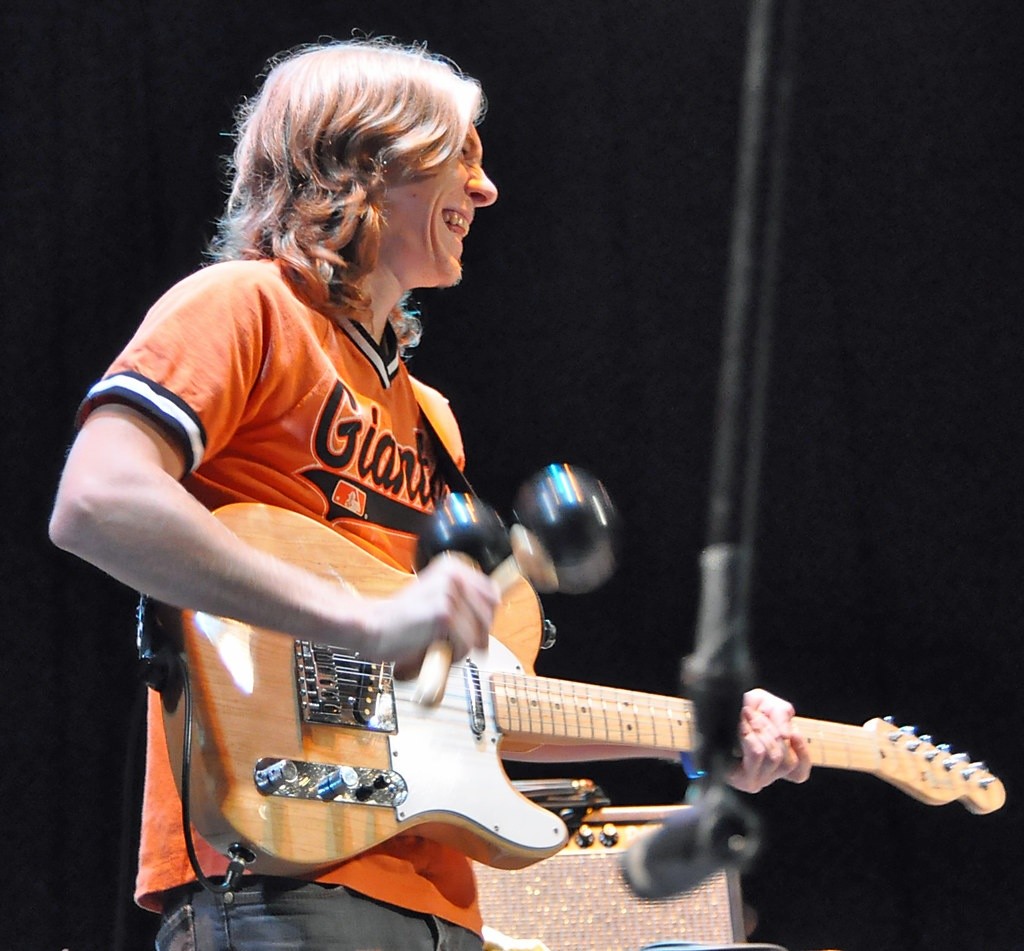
[48,42,812,950]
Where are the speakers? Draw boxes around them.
[471,806,799,951]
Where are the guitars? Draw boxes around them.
[157,499,1007,881]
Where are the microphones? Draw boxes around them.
[620,797,755,905]
